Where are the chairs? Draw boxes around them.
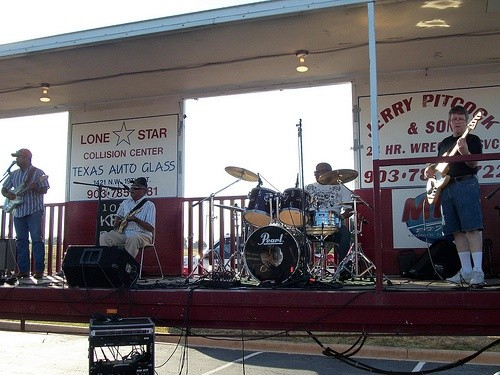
[117,227,164,280]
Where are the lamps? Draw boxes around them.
[296,51,309,72]
[39,84,50,102]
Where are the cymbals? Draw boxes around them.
[225,167,260,181]
[338,201,368,206]
[319,169,358,184]
[214,204,243,212]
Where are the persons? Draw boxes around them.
[1,149,50,279]
[424,105,485,284]
[100,177,156,273]
[305,162,354,280]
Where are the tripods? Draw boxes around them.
[185,173,244,283]
[271,123,393,289]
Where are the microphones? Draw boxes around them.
[298,119,303,137]
[3,161,17,176]
[120,182,130,190]
[257,173,262,185]
[295,174,299,188]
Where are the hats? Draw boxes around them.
[130,177,147,188]
[11,149,32,158]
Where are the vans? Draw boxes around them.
[194,235,242,275]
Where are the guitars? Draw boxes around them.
[426,111,482,205]
[4,175,48,213]
[118,208,142,232]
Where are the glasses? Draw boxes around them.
[131,185,145,190]
[449,118,467,122]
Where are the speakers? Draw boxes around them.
[61,245,140,286]
[406,240,462,279]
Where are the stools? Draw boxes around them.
[309,238,334,281]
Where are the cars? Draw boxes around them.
[184,256,196,276]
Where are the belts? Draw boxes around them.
[450,175,474,182]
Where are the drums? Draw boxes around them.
[278,188,310,227]
[243,225,312,284]
[306,208,341,235]
[243,188,279,226]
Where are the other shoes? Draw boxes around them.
[16,272,29,278]
[470,271,484,284]
[339,271,350,280]
[446,270,471,283]
[34,272,43,278]
[128,271,137,288]
